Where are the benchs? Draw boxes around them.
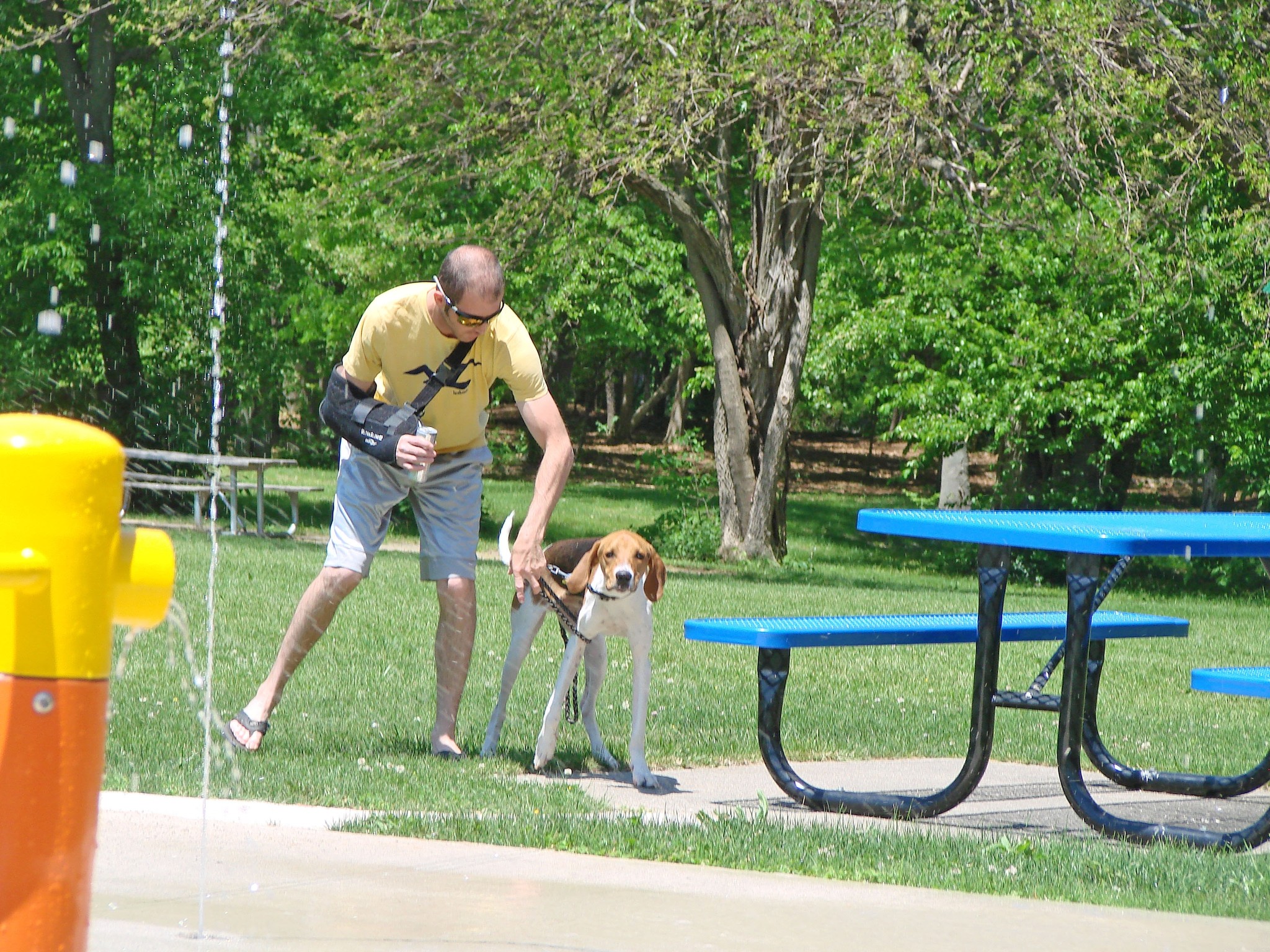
[1189,668,1270,842]
[681,610,1191,787]
[121,471,324,536]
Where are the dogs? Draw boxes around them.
[481,512,668,791]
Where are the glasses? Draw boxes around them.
[431,274,507,328]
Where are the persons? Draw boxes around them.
[225,245,575,760]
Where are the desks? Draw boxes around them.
[856,511,1270,824]
[123,448,297,537]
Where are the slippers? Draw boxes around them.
[222,708,270,754]
[430,750,468,763]
